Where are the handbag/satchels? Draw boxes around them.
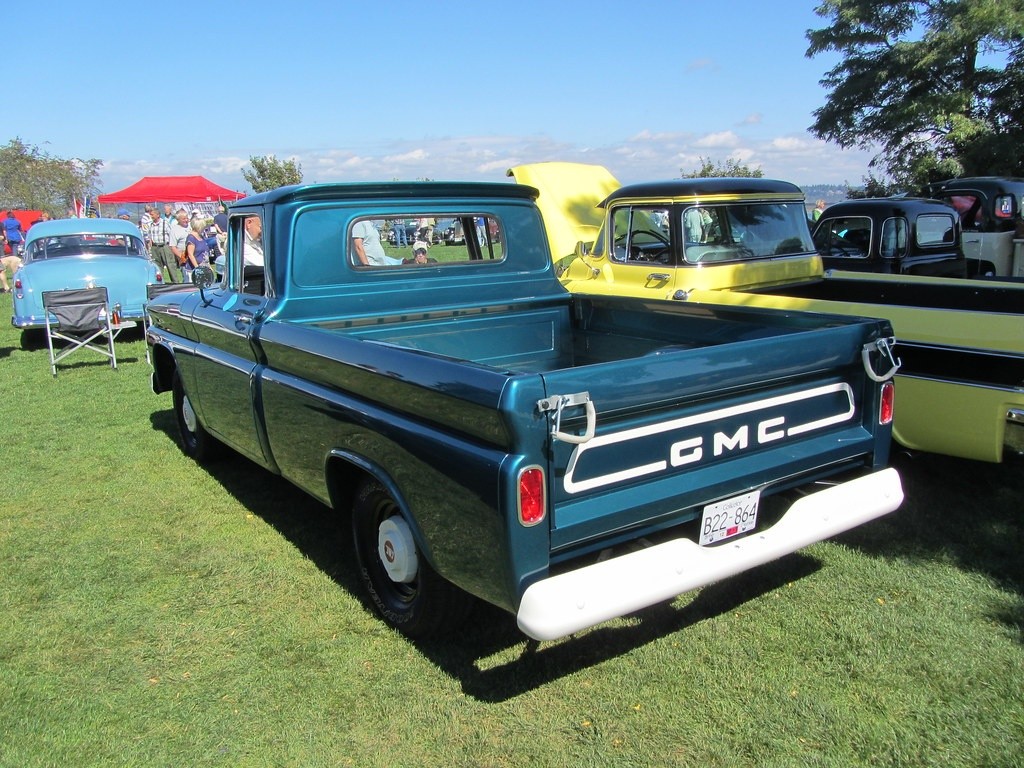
[4,243,12,255]
[179,249,188,266]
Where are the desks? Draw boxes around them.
[110,320,137,338]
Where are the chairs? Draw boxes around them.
[42,286,116,378]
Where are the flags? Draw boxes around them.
[74,195,100,218]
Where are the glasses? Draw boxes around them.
[414,250,426,255]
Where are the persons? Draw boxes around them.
[407,241,438,264]
[245,217,265,267]
[812,200,824,221]
[0,207,23,257]
[414,218,434,249]
[475,216,488,248]
[1002,201,1009,210]
[115,208,132,246]
[650,209,669,227]
[349,220,386,266]
[214,206,228,254]
[68,209,78,219]
[394,219,408,249]
[39,212,60,250]
[685,208,713,243]
[141,203,210,284]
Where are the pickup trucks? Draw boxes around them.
[140,179,906,641]
[509,161,1024,464]
[812,175,1024,283]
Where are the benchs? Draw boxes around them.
[687,237,805,265]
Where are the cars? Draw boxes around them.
[9,218,166,331]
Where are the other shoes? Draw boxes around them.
[4,287,12,294]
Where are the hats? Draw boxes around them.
[118,209,132,217]
[413,241,427,252]
[192,208,201,216]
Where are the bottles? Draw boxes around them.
[112,302,121,324]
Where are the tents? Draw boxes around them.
[98,176,247,218]
